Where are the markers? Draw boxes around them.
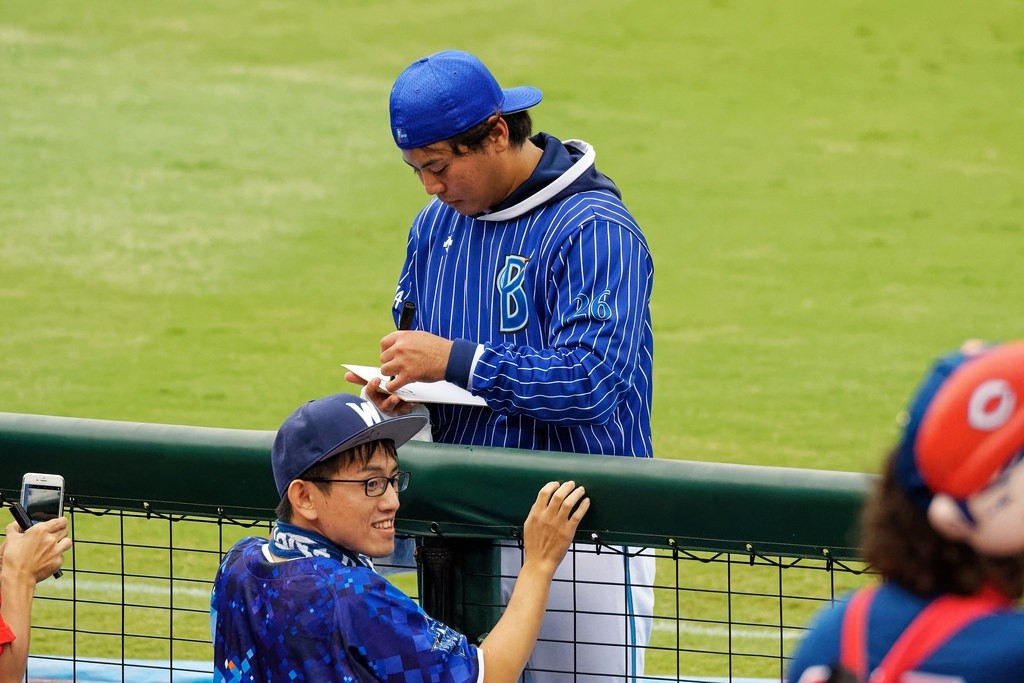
[8,502,64,579]
[389,300,417,382]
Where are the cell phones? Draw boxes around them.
[19,473,65,532]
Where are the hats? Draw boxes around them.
[389,49,543,150]
[271,393,428,500]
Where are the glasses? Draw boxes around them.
[299,471,411,497]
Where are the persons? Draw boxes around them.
[340,48,660,683]
[208,389,592,683]
[0,515,72,683]
[783,335,1024,683]
